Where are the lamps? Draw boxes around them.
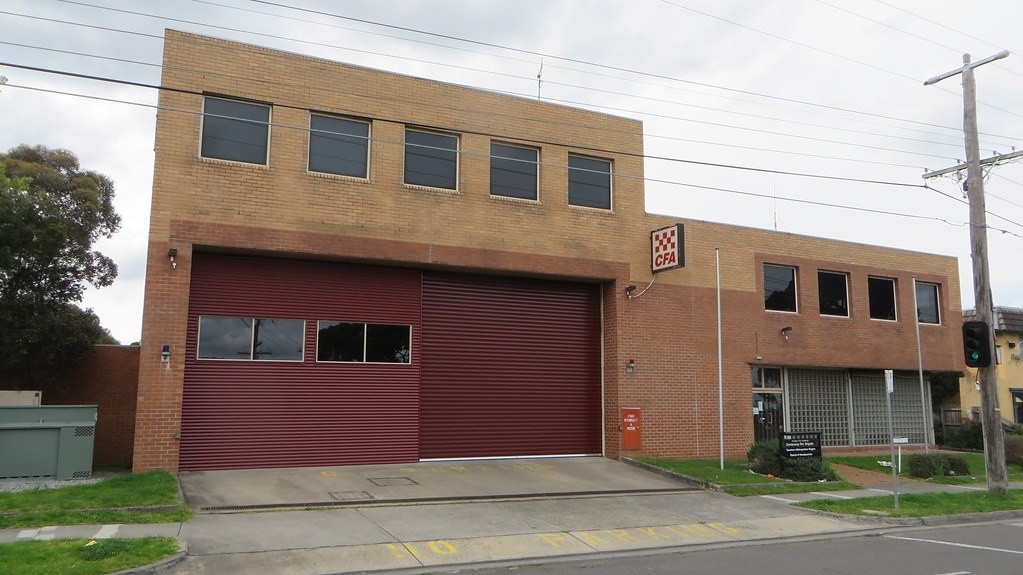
[625,284,637,291]
[782,326,792,336]
[161,344,171,363]
[627,359,635,372]
[168,248,178,261]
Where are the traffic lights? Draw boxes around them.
[962,321,992,367]
